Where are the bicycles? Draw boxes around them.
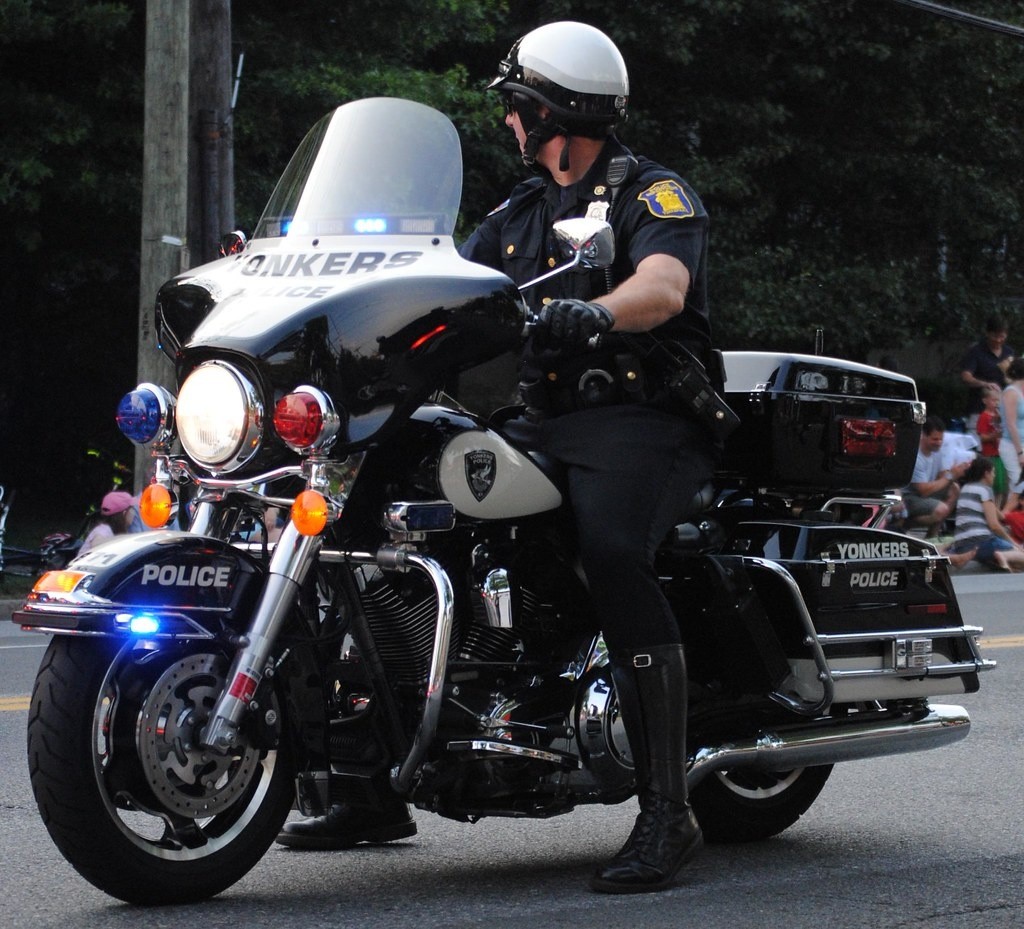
[78,449,134,538]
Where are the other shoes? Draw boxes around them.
[954,550,976,568]
[925,533,942,545]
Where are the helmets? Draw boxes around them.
[486,20,629,123]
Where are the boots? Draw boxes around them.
[589,643,704,893]
[276,779,417,847]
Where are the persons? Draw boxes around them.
[275,17,728,895]
[76,489,285,560]
[901,309,1024,571]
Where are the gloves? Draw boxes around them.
[529,297,617,359]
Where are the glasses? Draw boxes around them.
[504,99,517,114]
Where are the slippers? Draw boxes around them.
[986,561,1011,573]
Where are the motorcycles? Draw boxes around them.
[12,95,999,906]
